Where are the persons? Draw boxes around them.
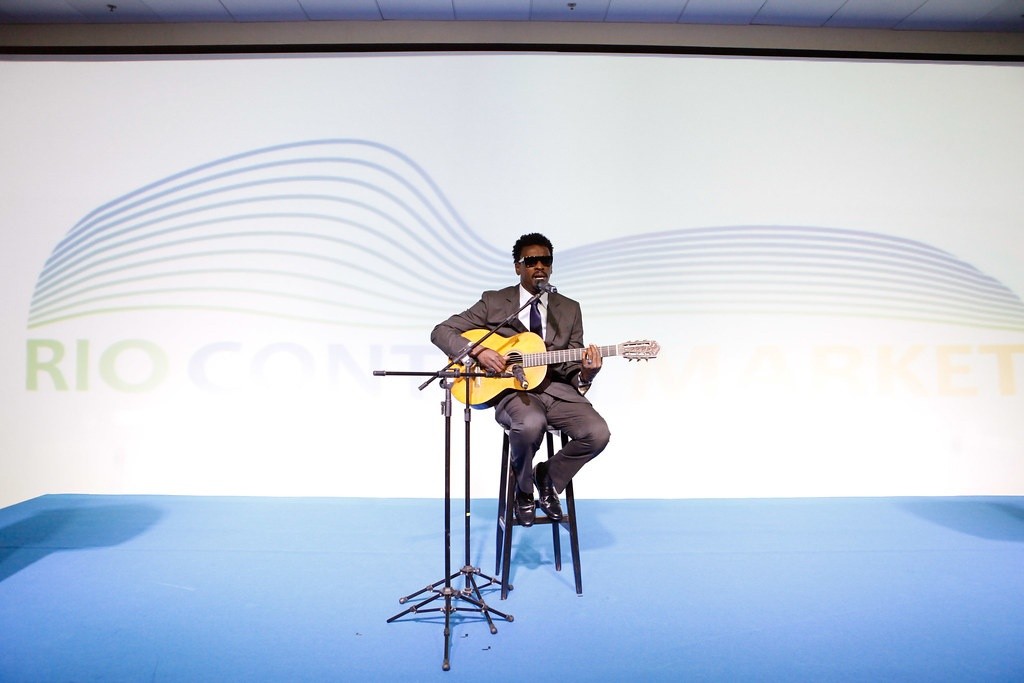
[430,233,611,527]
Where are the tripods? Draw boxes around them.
[372,289,545,672]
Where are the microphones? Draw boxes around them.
[512,365,529,390]
[537,279,558,294]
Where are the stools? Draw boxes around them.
[496,424,583,601]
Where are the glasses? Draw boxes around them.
[518,256,553,268]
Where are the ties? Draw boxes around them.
[528,297,543,339]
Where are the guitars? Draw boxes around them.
[448,329,660,410]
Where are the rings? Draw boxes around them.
[588,361,592,364]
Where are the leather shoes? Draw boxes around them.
[531,462,563,519]
[513,477,536,527]
